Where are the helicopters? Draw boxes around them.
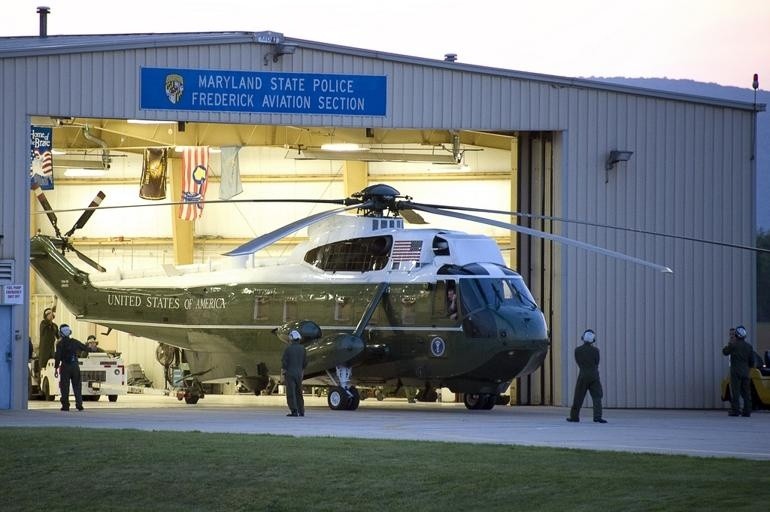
[30,177,770,411]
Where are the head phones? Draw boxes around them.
[85,335,98,346]
[43,307,55,318]
[58,323,72,336]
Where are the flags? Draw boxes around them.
[178,145,210,219]
[29,127,54,190]
[138,149,168,200]
[217,147,244,200]
[392,241,422,269]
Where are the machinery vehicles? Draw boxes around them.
[29,344,130,404]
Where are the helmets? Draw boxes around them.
[44,311,99,343]
[289,330,302,341]
[735,326,746,338]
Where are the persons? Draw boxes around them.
[281,330,308,416]
[447,289,461,320]
[27,295,103,412]
[723,325,758,417]
[729,328,736,342]
[566,329,608,424]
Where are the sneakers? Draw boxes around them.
[76,405,83,410]
[741,412,751,417]
[567,417,579,422]
[287,409,297,416]
[594,418,607,423]
[299,413,304,416]
[61,406,70,411]
[728,412,740,416]
